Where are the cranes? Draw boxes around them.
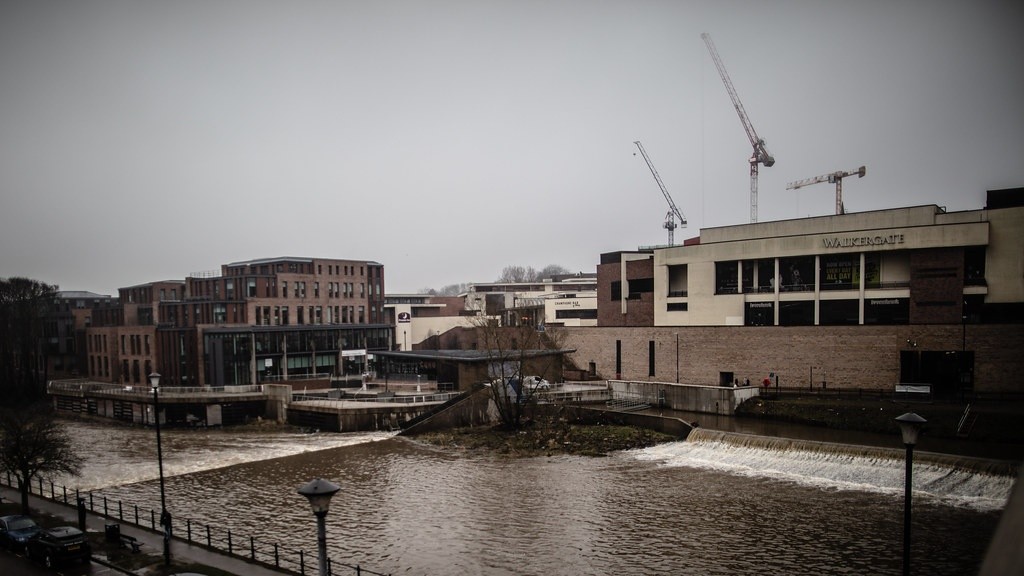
[629,137,687,249]
[700,31,777,224]
[783,164,866,215]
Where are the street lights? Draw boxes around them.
[143,372,175,521]
[295,474,340,575]
[891,409,928,573]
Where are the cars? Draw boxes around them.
[0,513,43,559]
[32,525,93,569]
